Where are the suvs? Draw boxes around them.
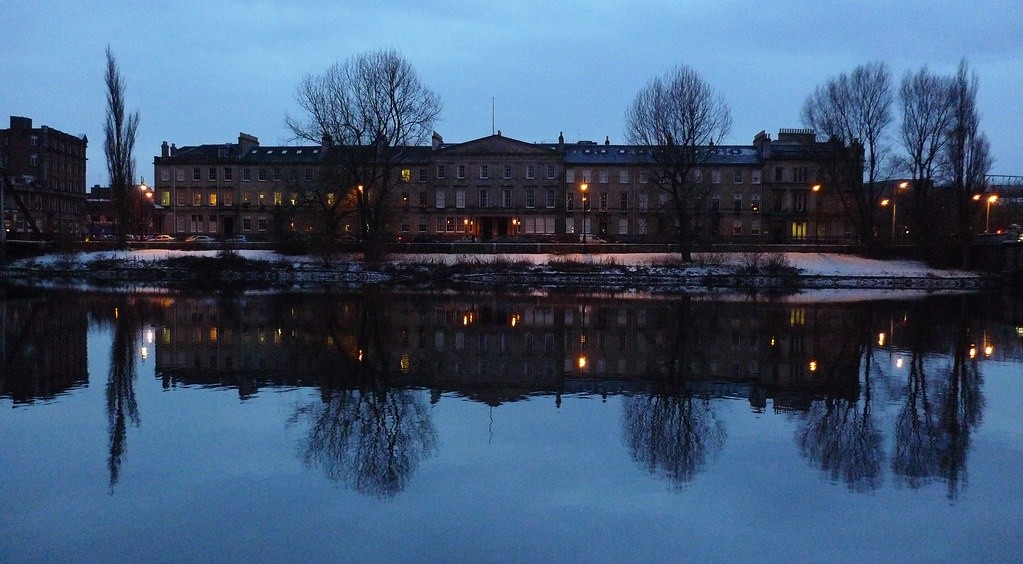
[225,235,250,242]
[529,234,564,243]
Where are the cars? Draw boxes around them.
[453,236,481,243]
[998,224,1023,237]
[99,234,147,241]
[339,234,364,243]
[148,235,176,242]
[185,235,216,242]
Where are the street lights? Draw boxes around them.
[579,302,586,372]
[968,329,993,359]
[810,310,817,372]
[814,182,821,243]
[581,176,588,243]
[141,175,147,241]
[893,181,907,239]
[974,193,995,234]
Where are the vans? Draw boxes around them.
[580,234,606,243]
